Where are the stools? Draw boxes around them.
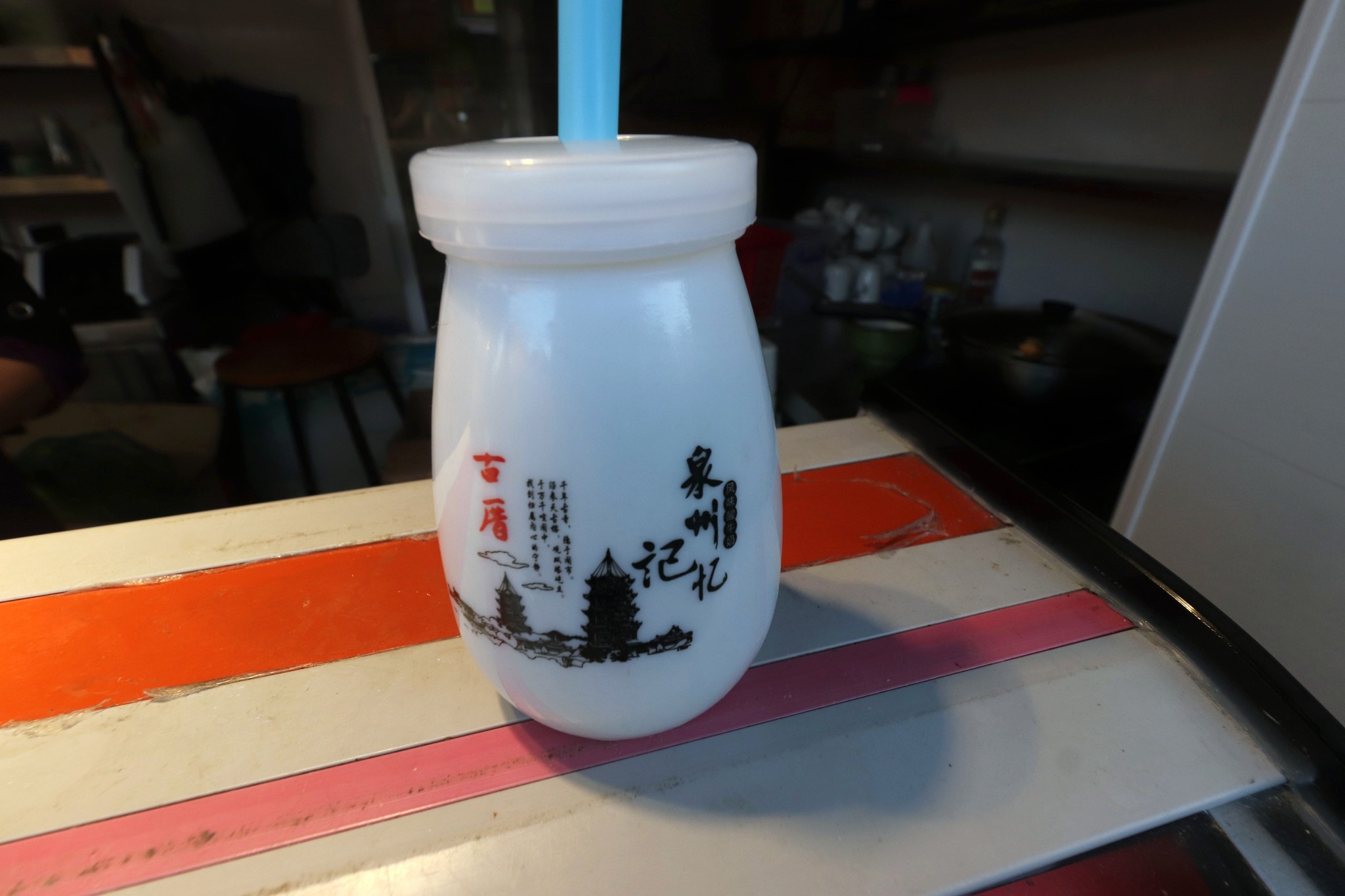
[213,309,421,491]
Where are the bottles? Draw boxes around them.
[406,133,785,741]
[784,194,1006,377]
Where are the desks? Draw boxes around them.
[0,383,1345,896]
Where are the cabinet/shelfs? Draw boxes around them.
[0,31,188,315]
